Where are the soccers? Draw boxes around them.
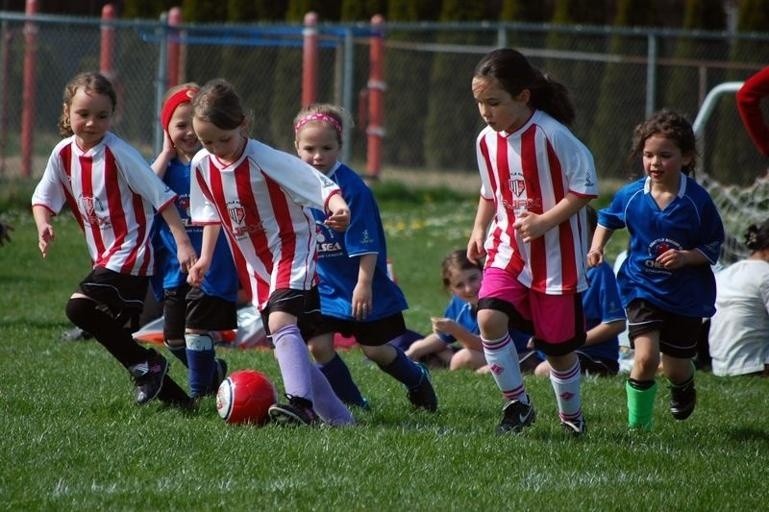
[216,369,275,423]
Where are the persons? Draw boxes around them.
[28,71,198,416]
[388,248,532,374]
[476,203,627,377]
[184,78,356,428]
[149,82,239,400]
[466,45,600,439]
[707,216,769,378]
[584,106,726,430]
[290,101,439,415]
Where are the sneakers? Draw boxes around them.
[214,358,227,394]
[406,361,437,414]
[560,418,587,437]
[360,397,371,411]
[665,360,697,422]
[155,395,196,411]
[496,395,535,435]
[267,394,319,427]
[130,348,168,407]
